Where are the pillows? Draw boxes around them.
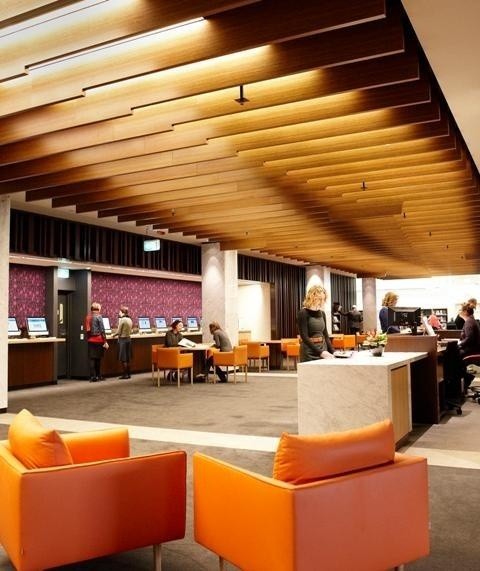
[272,419,396,485]
[7,408,73,470]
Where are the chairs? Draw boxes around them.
[150,332,375,389]
[439,341,480,416]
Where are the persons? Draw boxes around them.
[85,301,109,382]
[457,302,479,387]
[337,303,362,335]
[197,321,232,382]
[454,298,477,328]
[110,307,135,379]
[379,292,399,331]
[164,321,185,382]
[296,283,339,362]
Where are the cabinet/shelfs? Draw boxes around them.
[420,309,448,330]
[331,302,341,334]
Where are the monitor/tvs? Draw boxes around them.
[171,317,184,331]
[8,317,21,336]
[155,316,169,331]
[138,317,152,332]
[186,316,199,331]
[26,317,49,336]
[102,317,112,333]
[388,306,421,326]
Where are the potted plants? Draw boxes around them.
[363,331,389,357]
